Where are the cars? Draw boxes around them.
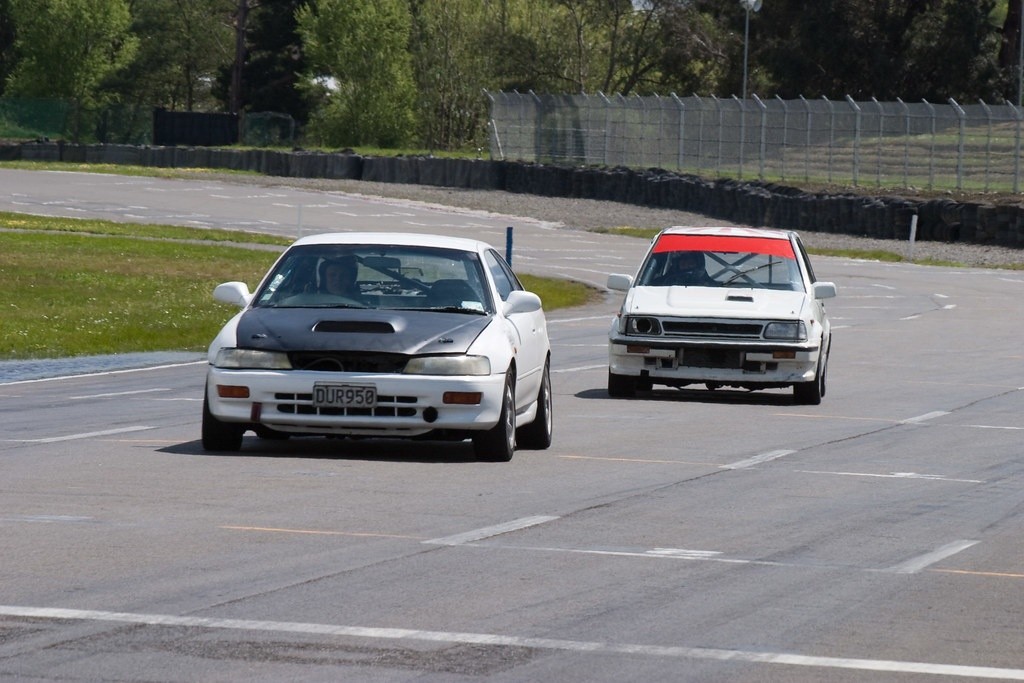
[200,231,558,462]
[602,227,838,405]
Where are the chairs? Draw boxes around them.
[422,280,478,308]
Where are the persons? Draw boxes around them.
[308,254,363,303]
[651,250,723,285]
